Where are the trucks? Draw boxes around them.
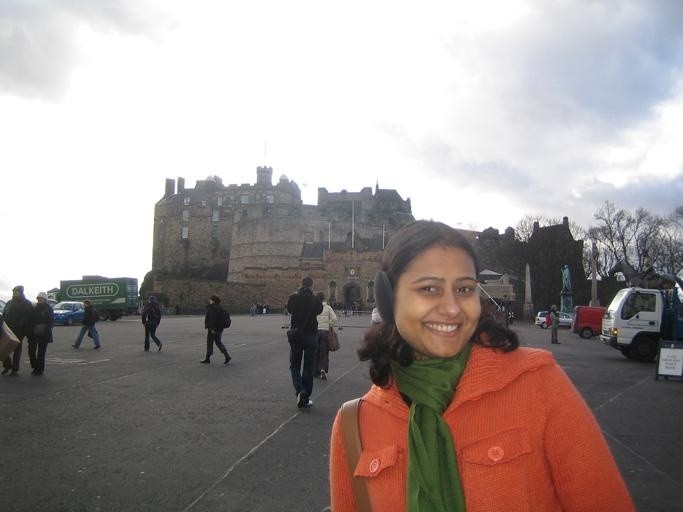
[570,305,608,340]
[598,283,683,365]
[46,277,140,322]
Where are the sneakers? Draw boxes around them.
[296,391,306,407]
[72,344,78,349]
[1,368,9,374]
[8,371,16,376]
[159,344,162,351]
[321,369,327,379]
[94,346,100,349]
[31,368,43,376]
[306,399,314,405]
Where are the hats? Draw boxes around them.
[210,294,221,302]
[37,292,48,301]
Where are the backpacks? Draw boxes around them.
[95,309,99,321]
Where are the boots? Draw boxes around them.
[201,354,210,362]
[224,351,231,364]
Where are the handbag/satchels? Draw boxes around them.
[32,324,52,339]
[223,311,231,328]
[88,330,97,337]
[287,328,306,344]
[328,333,340,351]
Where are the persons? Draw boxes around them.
[285,276,323,408]
[508,309,514,324]
[315,291,337,380]
[0,285,32,375]
[71,299,101,350]
[328,219,638,511]
[549,304,561,344]
[200,294,231,364]
[331,303,362,316]
[141,296,162,352]
[250,302,270,317]
[26,292,55,375]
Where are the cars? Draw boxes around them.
[50,300,84,324]
[533,310,573,330]
